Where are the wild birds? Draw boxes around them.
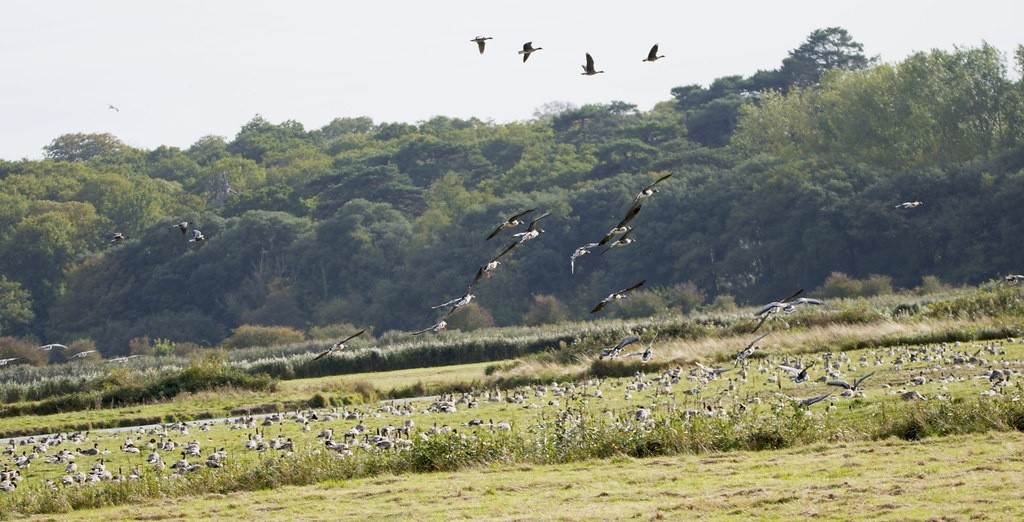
[581,53,603,75]
[470,209,552,288]
[470,35,493,54]
[0,273,1024,522]
[643,43,664,62]
[111,221,208,246]
[570,173,675,273]
[894,199,923,209]
[518,42,542,63]
